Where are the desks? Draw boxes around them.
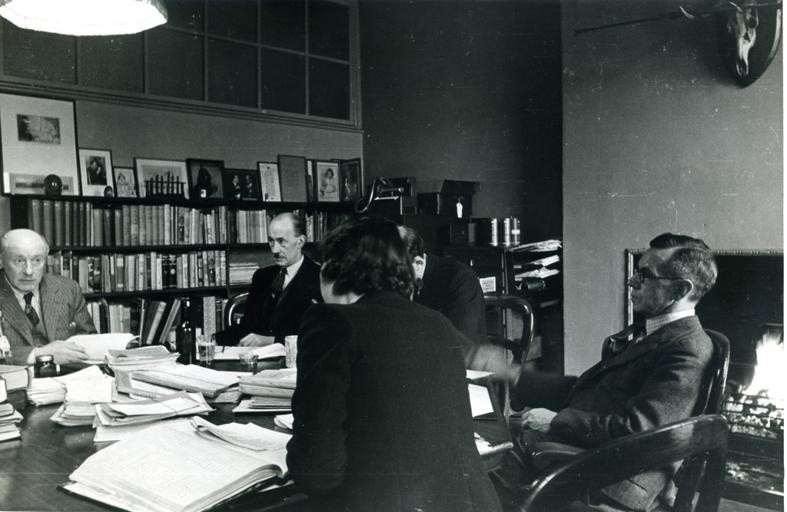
[0,359,525,510]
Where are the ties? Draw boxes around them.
[24,291,40,329]
[265,268,289,310]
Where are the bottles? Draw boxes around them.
[176,297,195,364]
[489,215,520,247]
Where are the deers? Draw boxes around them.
[679,1,759,80]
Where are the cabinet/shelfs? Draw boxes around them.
[7,191,395,351]
[455,238,563,369]
[396,211,506,259]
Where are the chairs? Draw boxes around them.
[675,324,735,509]
[515,409,737,512]
[466,288,537,377]
[222,291,249,329]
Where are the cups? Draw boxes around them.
[196,334,217,365]
[285,334,297,366]
[197,186,208,200]
[239,354,260,366]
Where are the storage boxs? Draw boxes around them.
[416,173,482,216]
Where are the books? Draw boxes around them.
[26,202,271,246]
[0,332,299,512]
[304,212,327,243]
[47,247,259,332]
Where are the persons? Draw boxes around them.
[393,224,488,370]
[225,172,242,198]
[282,215,499,511]
[489,232,717,510]
[88,154,105,184]
[320,168,339,198]
[0,228,97,362]
[341,164,358,201]
[210,209,323,350]
[243,172,256,198]
